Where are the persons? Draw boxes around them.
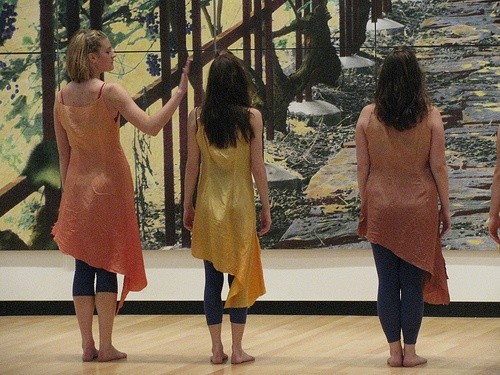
[487,126,500,245]
[53,31,194,364]
[183,53,272,365]
[355,46,452,368]
